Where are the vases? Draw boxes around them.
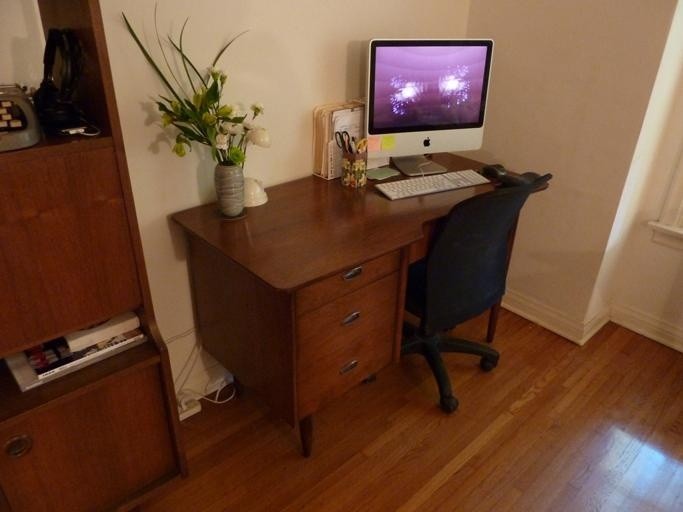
[214,162,245,218]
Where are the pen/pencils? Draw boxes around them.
[351,136,357,153]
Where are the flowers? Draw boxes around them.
[121,2,271,165]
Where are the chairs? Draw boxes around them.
[403,171,554,414]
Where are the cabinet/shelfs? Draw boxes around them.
[1,0,190,512]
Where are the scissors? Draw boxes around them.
[335,131,351,154]
[356,138,368,154]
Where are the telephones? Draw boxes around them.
[0,83,41,152]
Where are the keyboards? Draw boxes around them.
[374,169,491,201]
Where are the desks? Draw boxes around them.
[171,155,549,457]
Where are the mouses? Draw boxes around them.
[483,164,507,177]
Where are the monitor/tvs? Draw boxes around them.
[363,38,495,177]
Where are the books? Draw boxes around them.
[20,328,146,382]
[62,308,141,354]
[3,336,149,395]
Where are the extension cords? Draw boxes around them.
[177,398,202,421]
